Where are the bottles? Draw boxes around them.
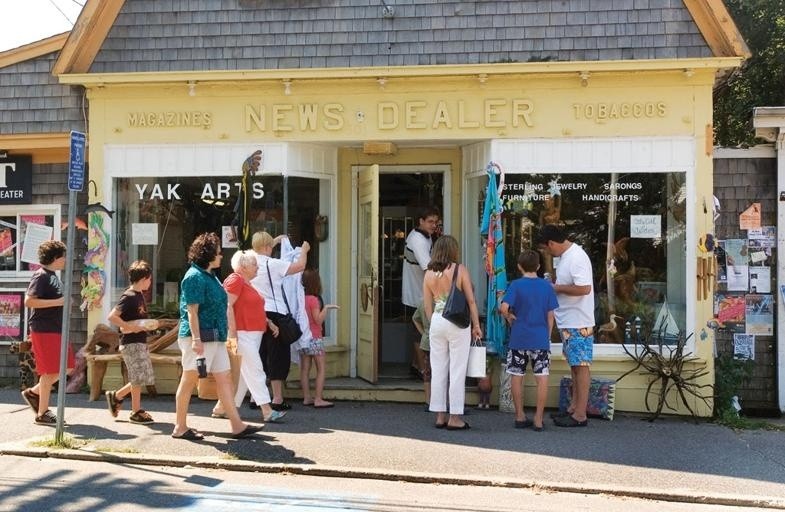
[196,354,208,378]
[542,272,553,282]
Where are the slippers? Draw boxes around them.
[232,424,266,439]
[211,412,230,419]
[270,398,292,411]
[550,408,587,427]
[514,415,545,431]
[263,410,288,423]
[303,399,334,408]
[435,422,471,430]
[249,402,260,409]
[172,428,204,441]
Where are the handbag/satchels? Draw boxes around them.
[466,346,486,378]
[277,313,303,345]
[197,354,241,400]
[442,286,470,329]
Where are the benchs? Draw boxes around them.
[82,319,183,402]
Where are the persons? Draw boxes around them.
[410,298,472,412]
[21,240,75,426]
[538,194,562,223]
[105,260,156,425]
[401,205,440,379]
[424,235,482,430]
[502,224,596,432]
[299,270,344,408]
[212,249,287,423]
[172,232,266,440]
[250,233,311,410]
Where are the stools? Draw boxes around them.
[559,375,616,420]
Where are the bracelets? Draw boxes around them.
[193,338,202,341]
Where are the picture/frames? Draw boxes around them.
[0,288,29,347]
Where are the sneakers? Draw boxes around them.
[105,389,124,417]
[128,408,154,425]
[21,387,67,426]
